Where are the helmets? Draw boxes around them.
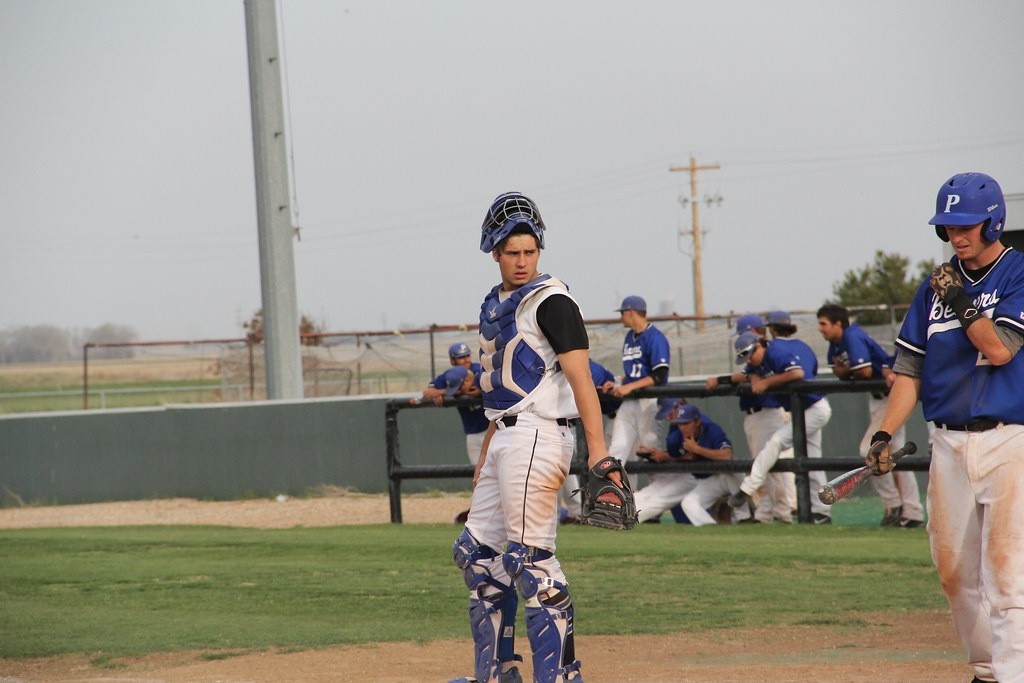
[928,172,1006,244]
[480,192,546,253]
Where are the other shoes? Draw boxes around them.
[727,489,749,508]
[737,518,761,525]
[810,512,832,528]
[880,505,924,529]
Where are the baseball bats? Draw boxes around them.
[816,440,919,504]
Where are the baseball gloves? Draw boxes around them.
[580,454,639,531]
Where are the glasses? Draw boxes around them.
[620,310,624,315]
[737,351,749,358]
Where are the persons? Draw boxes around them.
[558,295,752,527]
[865,172,1024,683]
[447,191,641,683]
[705,310,833,526]
[422,342,490,524]
[817,304,925,529]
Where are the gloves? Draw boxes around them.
[865,431,896,476]
[930,263,968,308]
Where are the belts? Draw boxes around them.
[494,416,575,430]
[746,407,762,415]
[933,419,1009,432]
[872,391,890,400]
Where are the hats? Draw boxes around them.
[729,314,762,337]
[734,331,759,365]
[673,404,699,423]
[655,397,681,420]
[615,296,646,311]
[762,311,791,325]
[445,366,468,395]
[449,343,471,358]
